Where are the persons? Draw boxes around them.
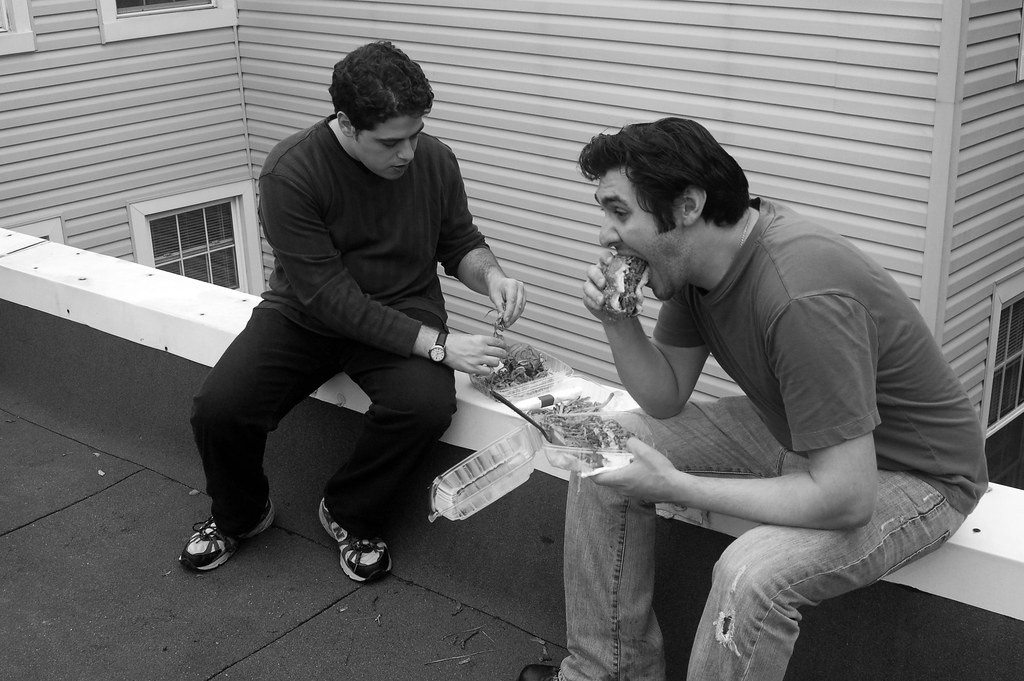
[178,40,527,582]
[521,117,990,681]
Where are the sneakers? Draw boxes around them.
[179,497,275,571]
[318,497,392,581]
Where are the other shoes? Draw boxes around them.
[515,664,559,681]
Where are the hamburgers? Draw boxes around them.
[598,252,650,320]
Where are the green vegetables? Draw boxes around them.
[482,309,551,387]
[524,394,633,450]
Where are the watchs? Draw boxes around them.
[428,331,448,363]
